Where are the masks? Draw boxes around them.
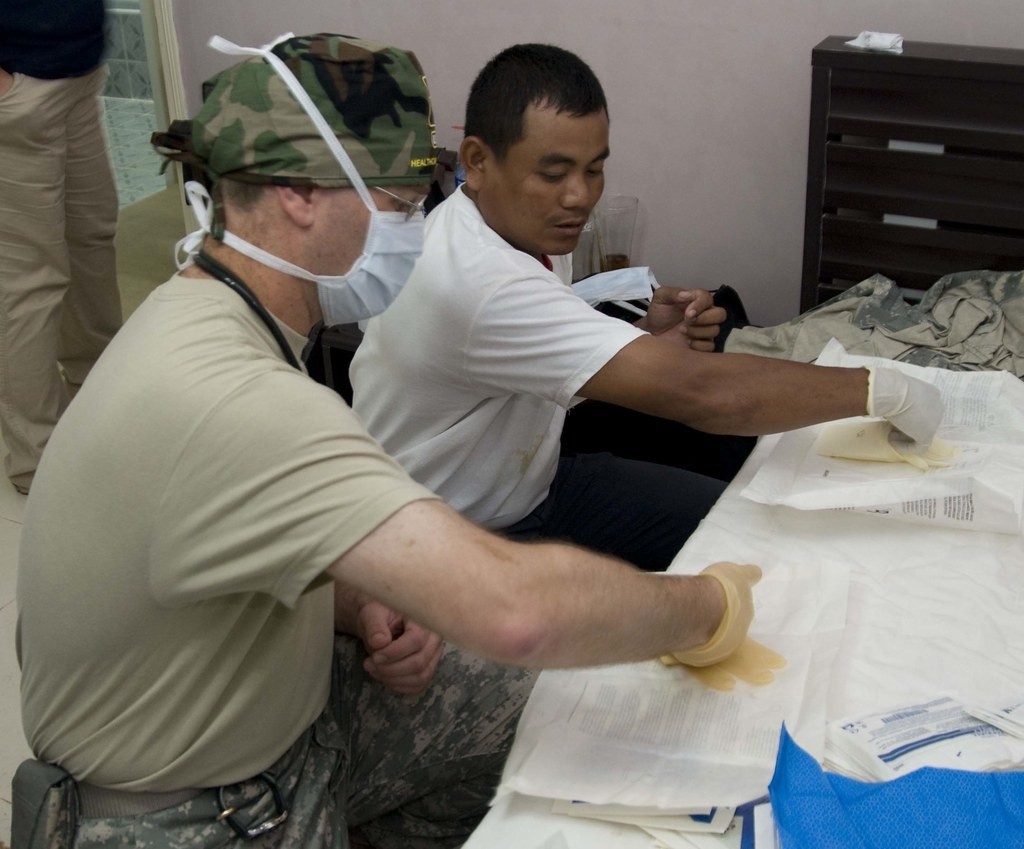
[273,147,425,327]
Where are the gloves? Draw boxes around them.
[660,561,786,693]
[816,422,959,470]
[862,364,946,457]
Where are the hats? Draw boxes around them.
[149,33,437,189]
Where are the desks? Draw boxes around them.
[460,431,1024,849]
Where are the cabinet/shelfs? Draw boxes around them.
[798,35,1024,316]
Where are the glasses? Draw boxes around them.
[372,181,431,227]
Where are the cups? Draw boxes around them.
[593,195,639,273]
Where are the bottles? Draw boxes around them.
[455,161,466,190]
[572,209,594,284]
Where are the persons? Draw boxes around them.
[14,34,762,849]
[0,0,121,497]
[348,44,945,572]
